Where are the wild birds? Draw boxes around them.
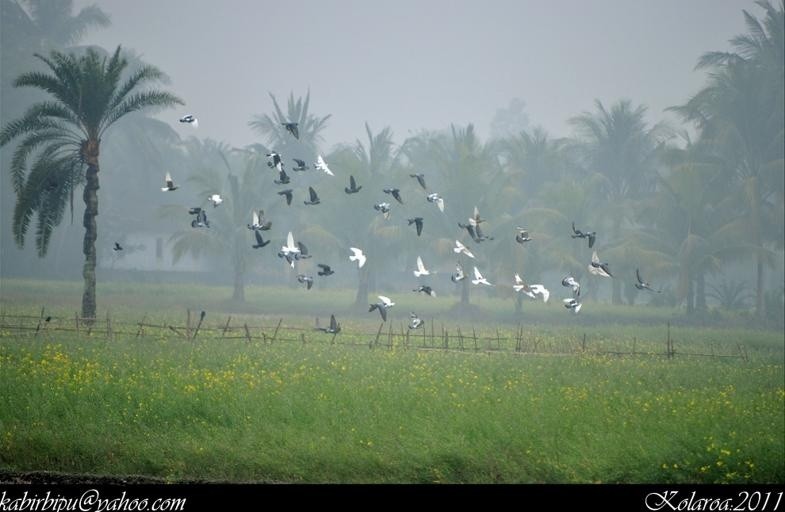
[179,114,197,125]
[245,150,496,334]
[282,122,299,140]
[570,223,612,277]
[187,192,224,230]
[515,227,531,243]
[113,241,123,251]
[634,268,661,293]
[513,271,582,314]
[160,171,181,192]
